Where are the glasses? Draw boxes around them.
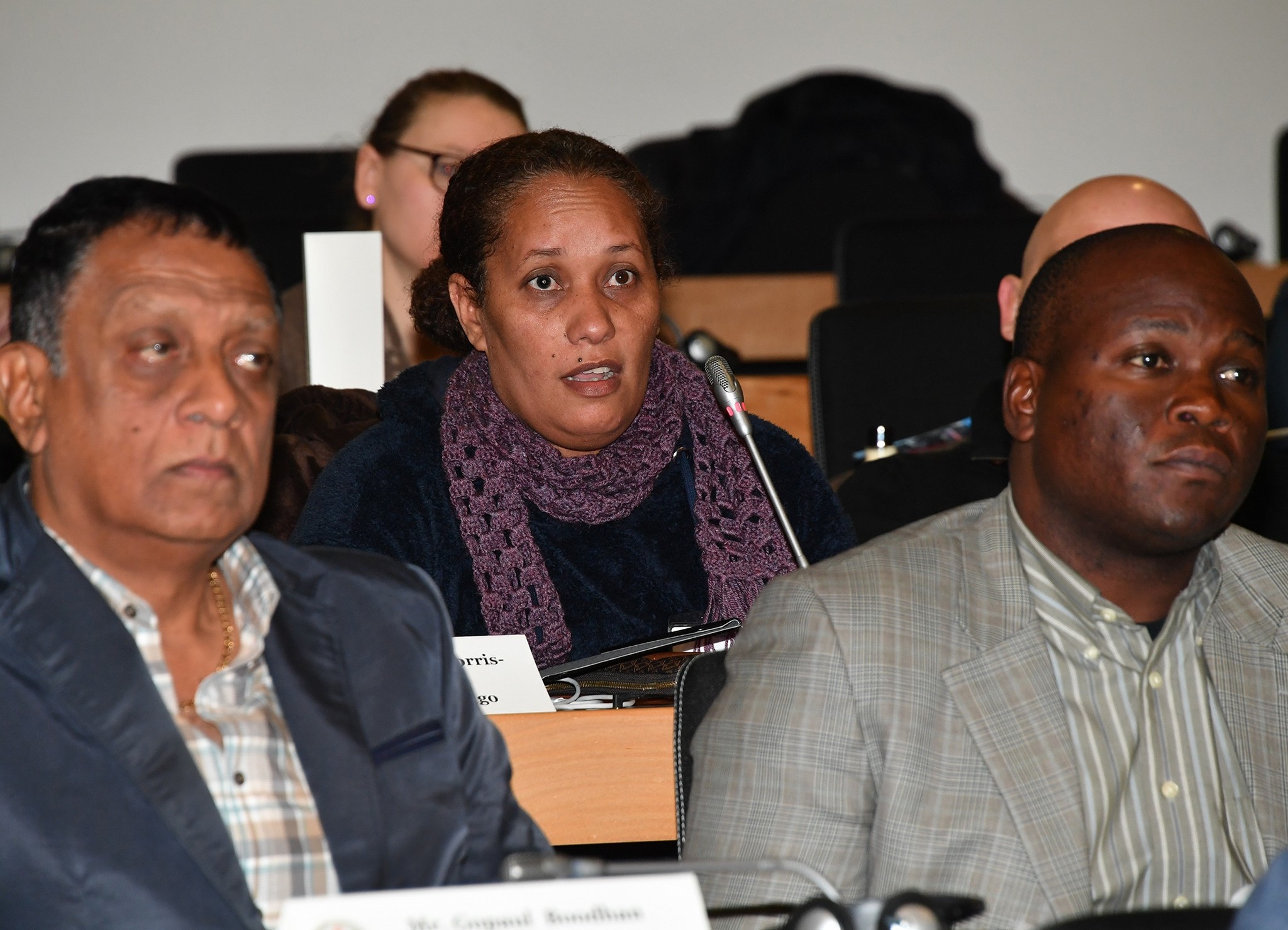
[391,140,462,195]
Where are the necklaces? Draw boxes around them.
[175,567,235,713]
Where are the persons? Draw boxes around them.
[284,129,856,661]
[807,174,1288,549]
[681,224,1288,929]
[280,69,532,400]
[1,179,564,928]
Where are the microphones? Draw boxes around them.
[704,353,812,570]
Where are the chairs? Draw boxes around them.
[804,294,1018,486]
[170,138,385,306]
